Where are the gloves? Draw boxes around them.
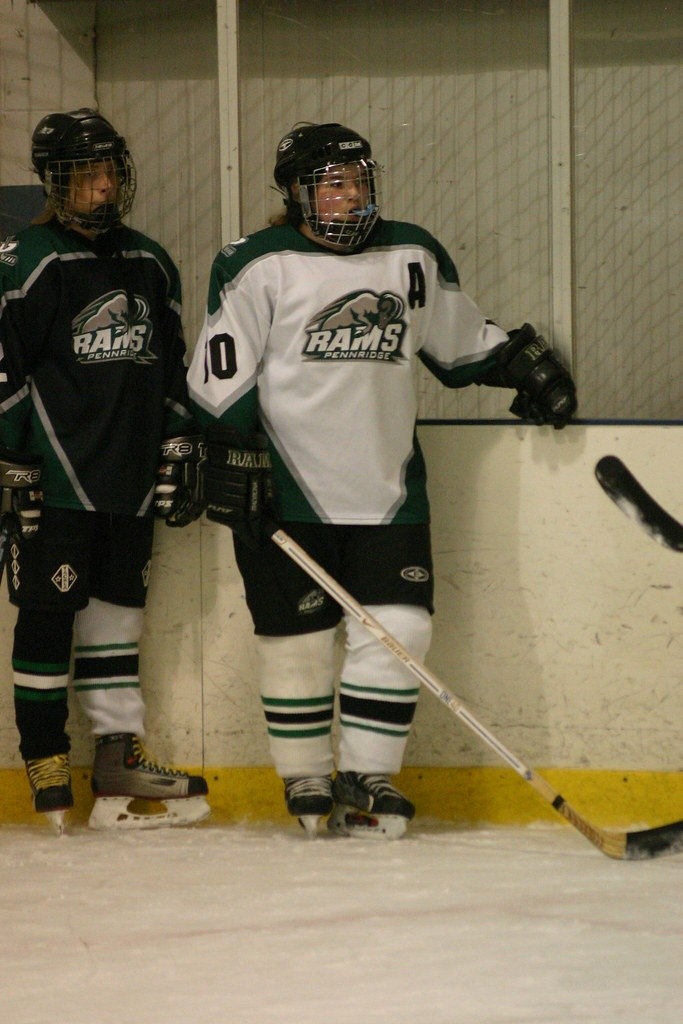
[496,324,578,431]
[154,435,208,529]
[203,426,279,533]
[0,445,44,545]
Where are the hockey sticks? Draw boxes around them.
[595,451,683,554]
[259,514,683,861]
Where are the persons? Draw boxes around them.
[0,107,212,811]
[186,123,577,818]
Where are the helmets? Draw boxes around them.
[273,123,378,247]
[31,108,137,229]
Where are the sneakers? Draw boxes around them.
[26,752,74,834]
[282,774,333,837]
[86,733,211,828]
[327,772,415,838]
